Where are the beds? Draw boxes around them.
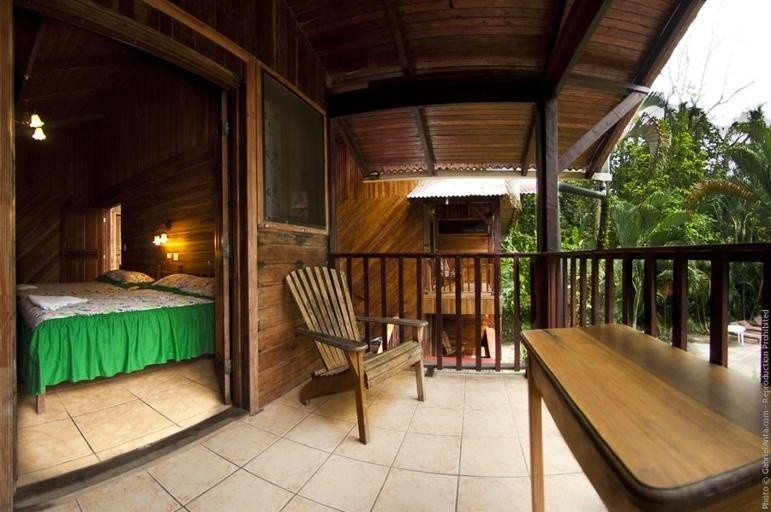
[16,259,216,415]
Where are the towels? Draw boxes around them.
[17,284,39,290]
[28,294,88,311]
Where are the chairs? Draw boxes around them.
[286,266,429,445]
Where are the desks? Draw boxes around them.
[727,325,746,345]
[520,323,771,511]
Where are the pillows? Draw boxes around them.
[96,270,156,289]
[142,273,216,300]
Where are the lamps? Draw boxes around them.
[153,221,170,246]
